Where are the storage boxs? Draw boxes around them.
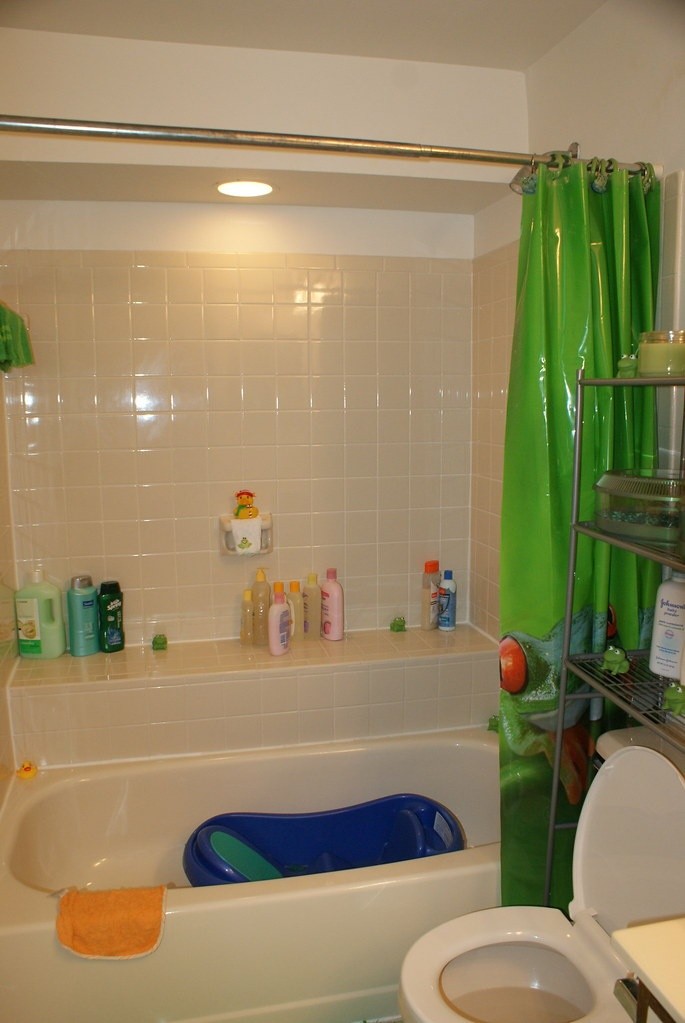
[593,470,685,548]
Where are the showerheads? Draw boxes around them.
[508,165,535,197]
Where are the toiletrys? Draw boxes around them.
[13,565,66,658]
[240,588,255,646]
[321,566,345,642]
[285,579,305,645]
[645,565,685,679]
[301,573,322,643]
[251,566,270,649]
[270,581,291,612]
[66,574,101,656]
[265,591,290,658]
[94,580,128,654]
[422,559,442,631]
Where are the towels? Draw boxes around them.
[0,299,36,374]
[231,516,264,558]
[53,881,181,962]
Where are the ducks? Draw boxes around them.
[232,489,259,519]
[15,761,37,779]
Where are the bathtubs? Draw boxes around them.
[0,725,505,1023]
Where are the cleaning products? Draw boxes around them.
[436,568,457,632]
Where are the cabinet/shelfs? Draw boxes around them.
[542,367,685,910]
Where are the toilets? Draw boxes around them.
[397,743,685,1023]
